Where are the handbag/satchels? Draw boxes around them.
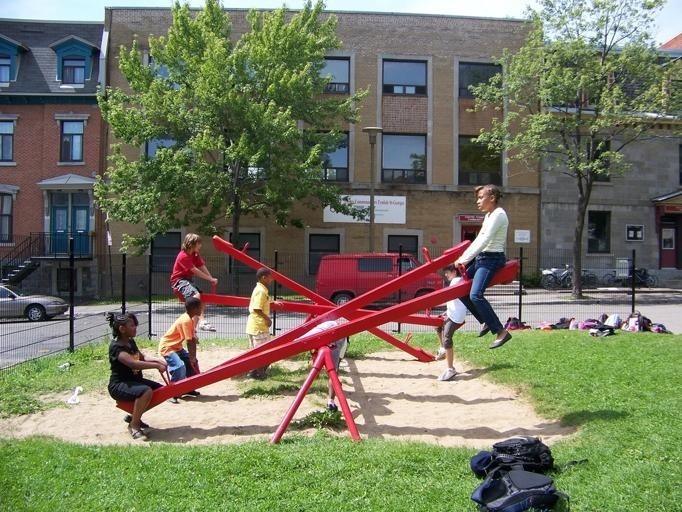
[620,310,666,333]
[505,317,530,330]
[550,313,622,338]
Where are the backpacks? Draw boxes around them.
[470,437,570,512]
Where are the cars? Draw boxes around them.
[0,283,71,322]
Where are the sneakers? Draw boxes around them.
[327,404,338,412]
[199,322,215,331]
[247,369,267,380]
[477,327,490,337]
[126,424,147,440]
[435,350,446,361]
[440,367,456,380]
[182,391,200,396]
[124,414,149,428]
[489,332,512,349]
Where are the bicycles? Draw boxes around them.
[539,262,661,289]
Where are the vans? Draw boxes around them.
[316,252,445,313]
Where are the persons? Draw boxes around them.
[453,185,513,349]
[242,266,284,383]
[430,263,468,381]
[169,231,218,347]
[158,297,202,405]
[102,311,168,440]
